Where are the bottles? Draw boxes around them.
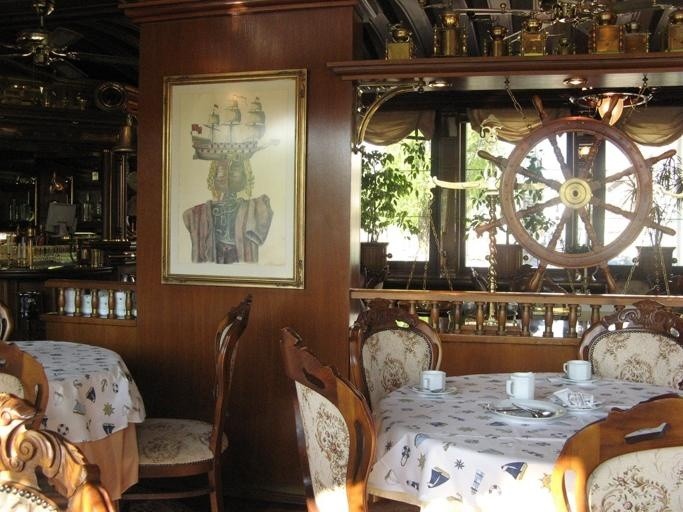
[80,194,100,220]
[7,198,26,219]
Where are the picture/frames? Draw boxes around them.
[160,67,310,292]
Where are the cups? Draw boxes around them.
[562,360,592,379]
[11,242,76,264]
[417,371,446,390]
[505,371,534,399]
[90,250,99,267]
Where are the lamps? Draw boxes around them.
[567,90,655,128]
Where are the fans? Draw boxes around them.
[1,2,138,73]
[452,0,631,48]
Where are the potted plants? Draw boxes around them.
[466,157,546,280]
[359,148,421,279]
[616,156,681,282]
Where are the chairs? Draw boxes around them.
[0,342,47,431]
[549,392,682,512]
[0,302,14,341]
[132,292,254,507]
[277,322,415,511]
[350,297,441,412]
[1,391,114,512]
[578,299,682,385]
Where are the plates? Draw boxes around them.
[411,384,455,396]
[483,399,566,421]
[564,375,599,384]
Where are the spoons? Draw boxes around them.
[512,402,551,417]
